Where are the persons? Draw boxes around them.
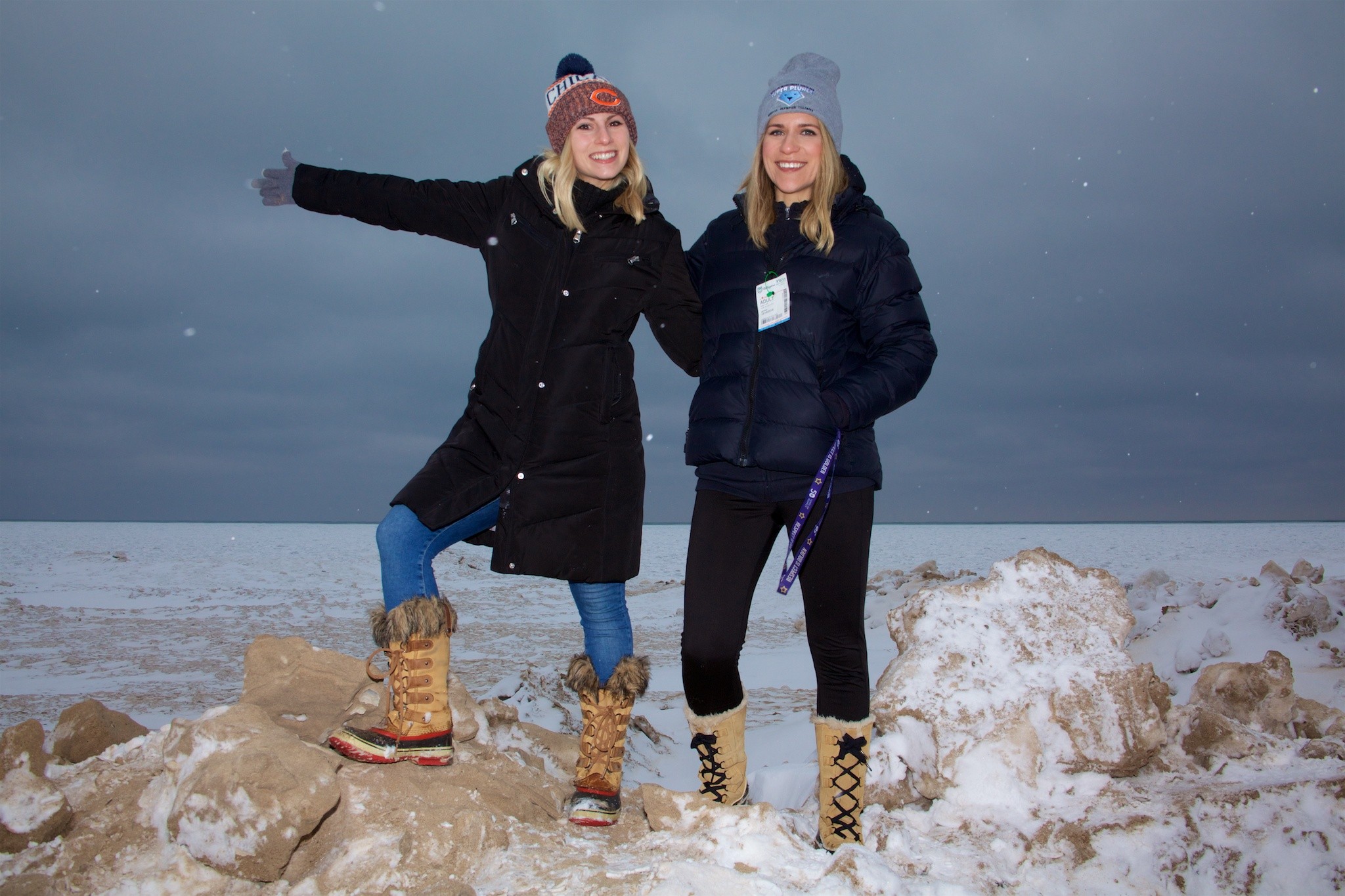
[670,78,944,852]
[251,75,709,843]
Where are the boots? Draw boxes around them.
[328,596,454,766]
[810,710,872,854]
[566,655,652,826]
[686,694,750,806]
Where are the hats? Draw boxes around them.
[756,52,842,156]
[544,52,638,154]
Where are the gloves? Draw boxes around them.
[251,148,302,206]
